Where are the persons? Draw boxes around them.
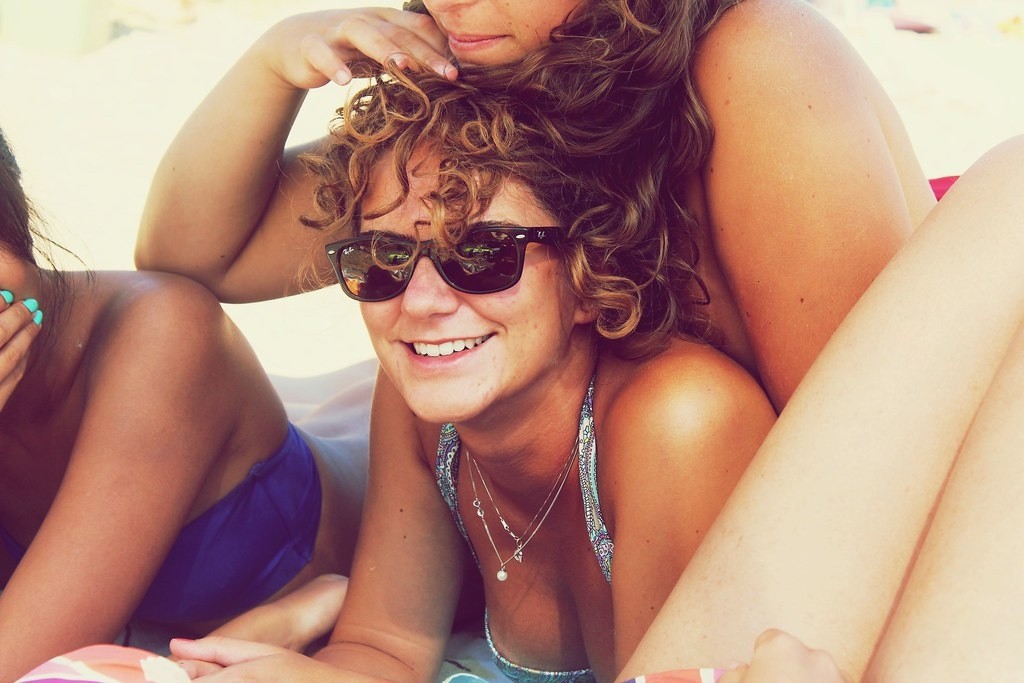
[2,0,1024,683]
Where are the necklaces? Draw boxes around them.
[472,435,578,564]
[466,444,581,581]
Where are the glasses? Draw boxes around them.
[325,226,567,302]
